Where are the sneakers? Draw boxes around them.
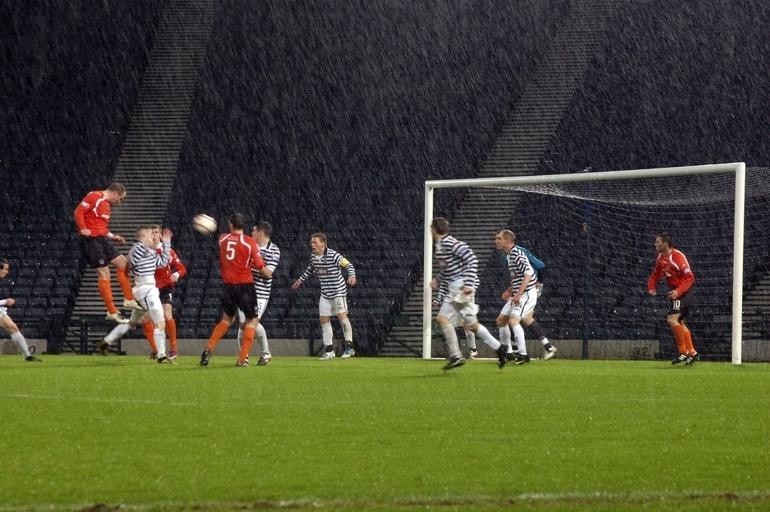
[26,356,43,361]
[236,352,271,366]
[465,347,478,357]
[105,312,130,324]
[200,350,210,367]
[97,339,126,355]
[151,350,176,363]
[122,299,145,311]
[443,356,465,370]
[670,351,698,365]
[319,351,335,362]
[496,342,529,368]
[543,345,556,361]
[340,348,355,359]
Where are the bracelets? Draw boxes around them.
[172,270,181,280]
[106,231,114,240]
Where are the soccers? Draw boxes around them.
[193,214,217,236]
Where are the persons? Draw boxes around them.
[647,230,699,367]
[503,227,559,363]
[431,275,482,360]
[72,181,143,325]
[235,219,283,366]
[140,225,187,363]
[99,223,180,366]
[429,215,515,371]
[0,256,44,362]
[494,226,540,367]
[289,230,359,360]
[198,211,264,368]
